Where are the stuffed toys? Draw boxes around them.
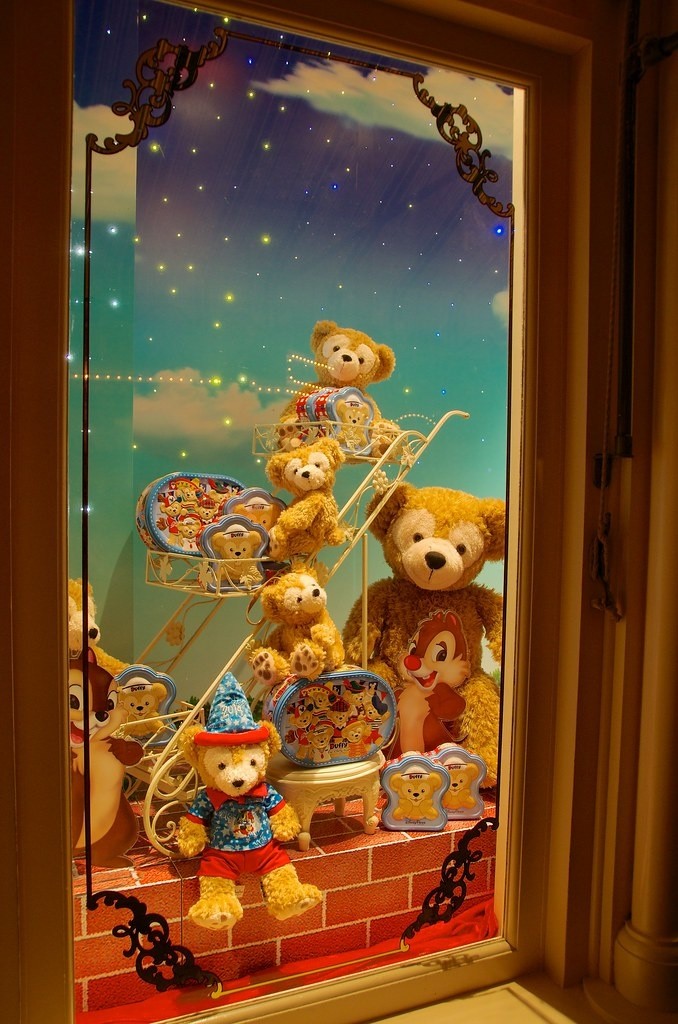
[68,319,507,931]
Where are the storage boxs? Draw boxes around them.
[136,472,246,557]
[262,665,397,769]
[379,751,451,831]
[315,386,374,456]
[296,386,332,442]
[114,664,176,746]
[219,488,288,557]
[196,513,269,593]
[425,742,487,820]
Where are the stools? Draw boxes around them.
[266,748,386,851]
